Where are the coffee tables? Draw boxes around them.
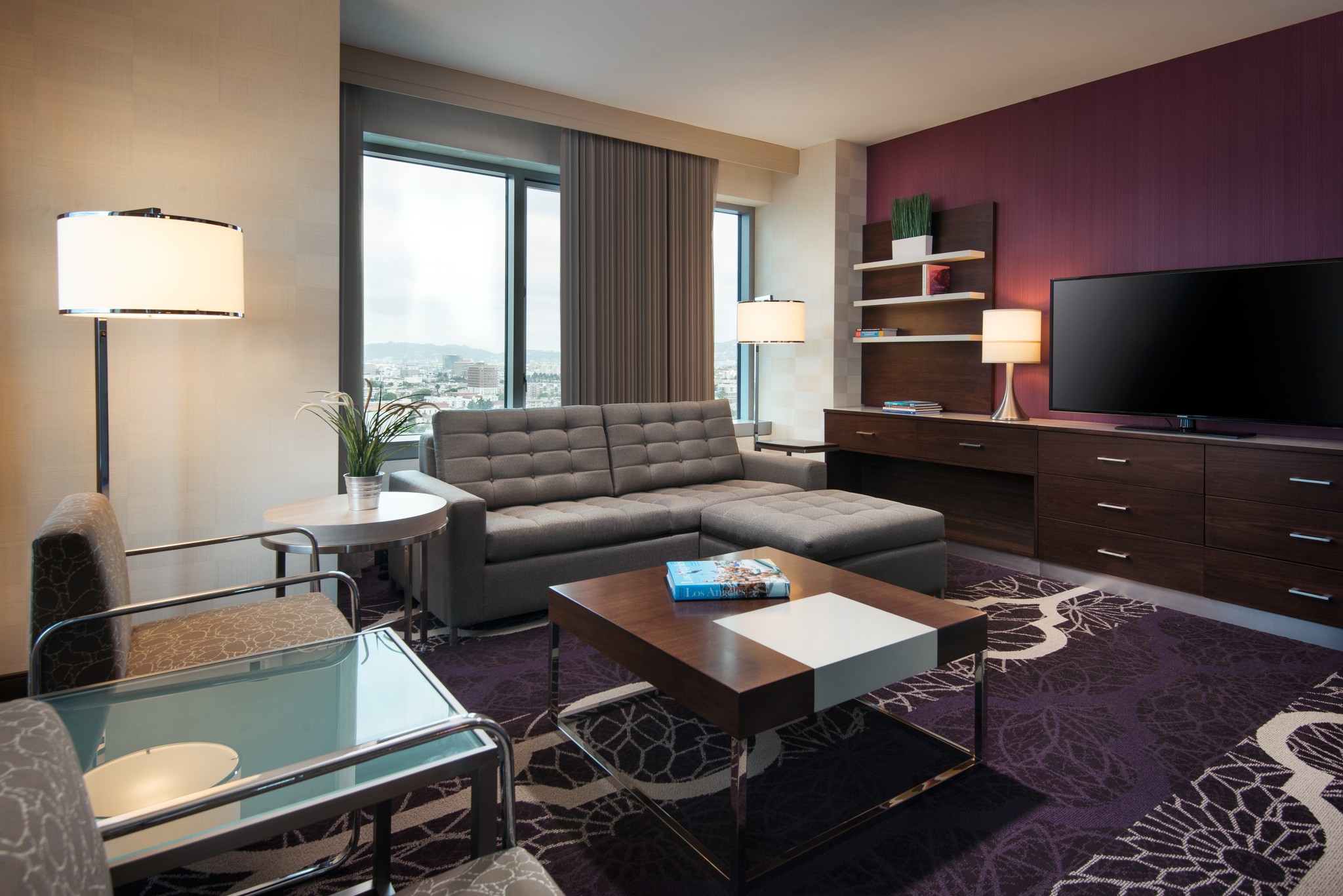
[546,538,987,896]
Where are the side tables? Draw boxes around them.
[32,626,499,896]
[755,439,839,487]
[258,493,449,648]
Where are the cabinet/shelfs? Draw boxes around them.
[851,250,989,343]
[822,408,1343,632]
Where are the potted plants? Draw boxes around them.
[892,191,934,259]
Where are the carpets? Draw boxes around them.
[114,554,1343,895]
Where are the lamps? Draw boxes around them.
[982,309,1042,422]
[54,206,246,495]
[734,295,807,443]
[82,742,244,870]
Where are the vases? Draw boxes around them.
[343,471,384,511]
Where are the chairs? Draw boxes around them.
[0,701,566,896]
[31,494,359,698]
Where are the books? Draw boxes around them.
[883,400,943,415]
[664,556,790,601]
[855,328,898,337]
[922,264,950,296]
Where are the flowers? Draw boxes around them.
[292,380,446,475]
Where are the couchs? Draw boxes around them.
[389,398,828,639]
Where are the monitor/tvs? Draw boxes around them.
[1049,258,1343,437]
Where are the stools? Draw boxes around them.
[699,488,947,605]
